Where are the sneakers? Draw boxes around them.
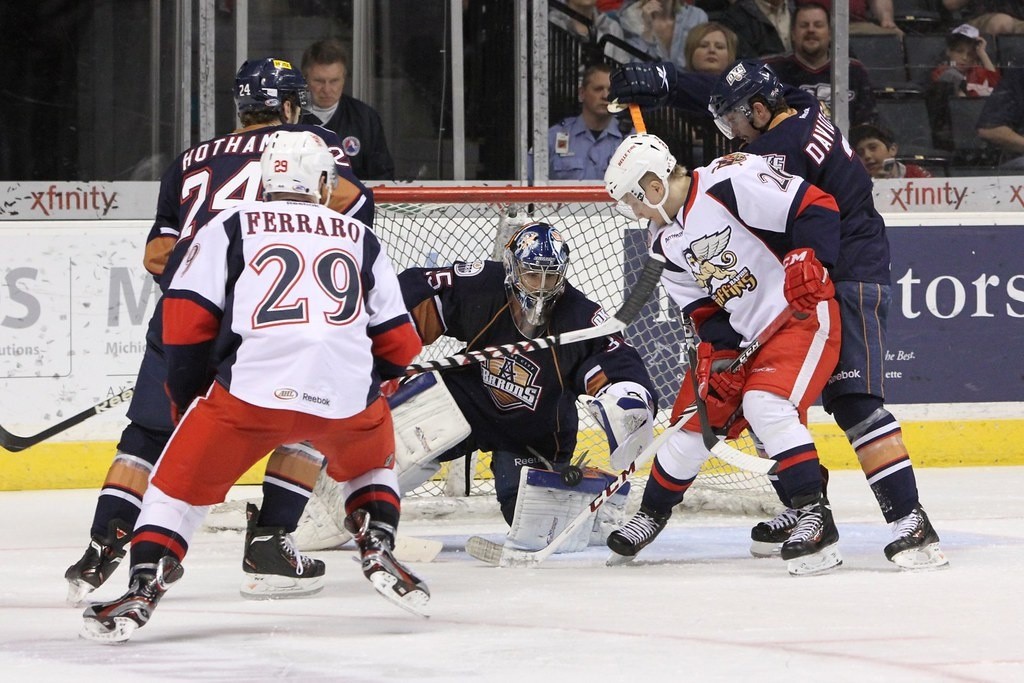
[243,502,325,599]
[751,509,799,557]
[884,509,950,570]
[346,511,431,619]
[80,557,183,644]
[64,519,133,605]
[781,504,843,573]
[605,509,674,566]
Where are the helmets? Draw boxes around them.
[234,57,308,114]
[505,222,570,324]
[709,59,786,122]
[605,133,677,208]
[260,131,336,201]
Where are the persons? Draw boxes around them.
[82,129,434,628]
[300,39,396,181]
[606,59,941,562]
[848,121,933,179]
[64,56,376,591]
[205,220,659,553]
[548,0,1024,173]
[527,63,637,181]
[602,131,841,561]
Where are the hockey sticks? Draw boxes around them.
[313,458,447,566]
[465,304,800,568]
[0,385,138,455]
[629,101,783,480]
[406,249,666,374]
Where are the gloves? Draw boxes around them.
[783,248,835,314]
[696,343,745,408]
[609,61,678,107]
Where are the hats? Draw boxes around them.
[946,24,983,42]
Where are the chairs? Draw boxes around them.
[850,0,1024,176]
